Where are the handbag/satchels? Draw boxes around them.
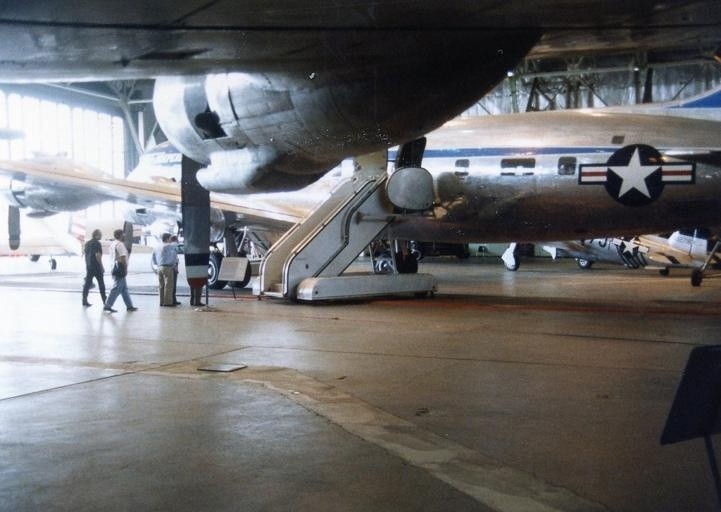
[111,262,126,277]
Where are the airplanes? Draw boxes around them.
[1,0,721,306]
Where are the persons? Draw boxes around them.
[170,235,186,305]
[102,229,140,312]
[152,233,179,306]
[82,227,108,307]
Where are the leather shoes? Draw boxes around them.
[83,302,138,312]
[160,302,181,306]
[191,303,205,306]
